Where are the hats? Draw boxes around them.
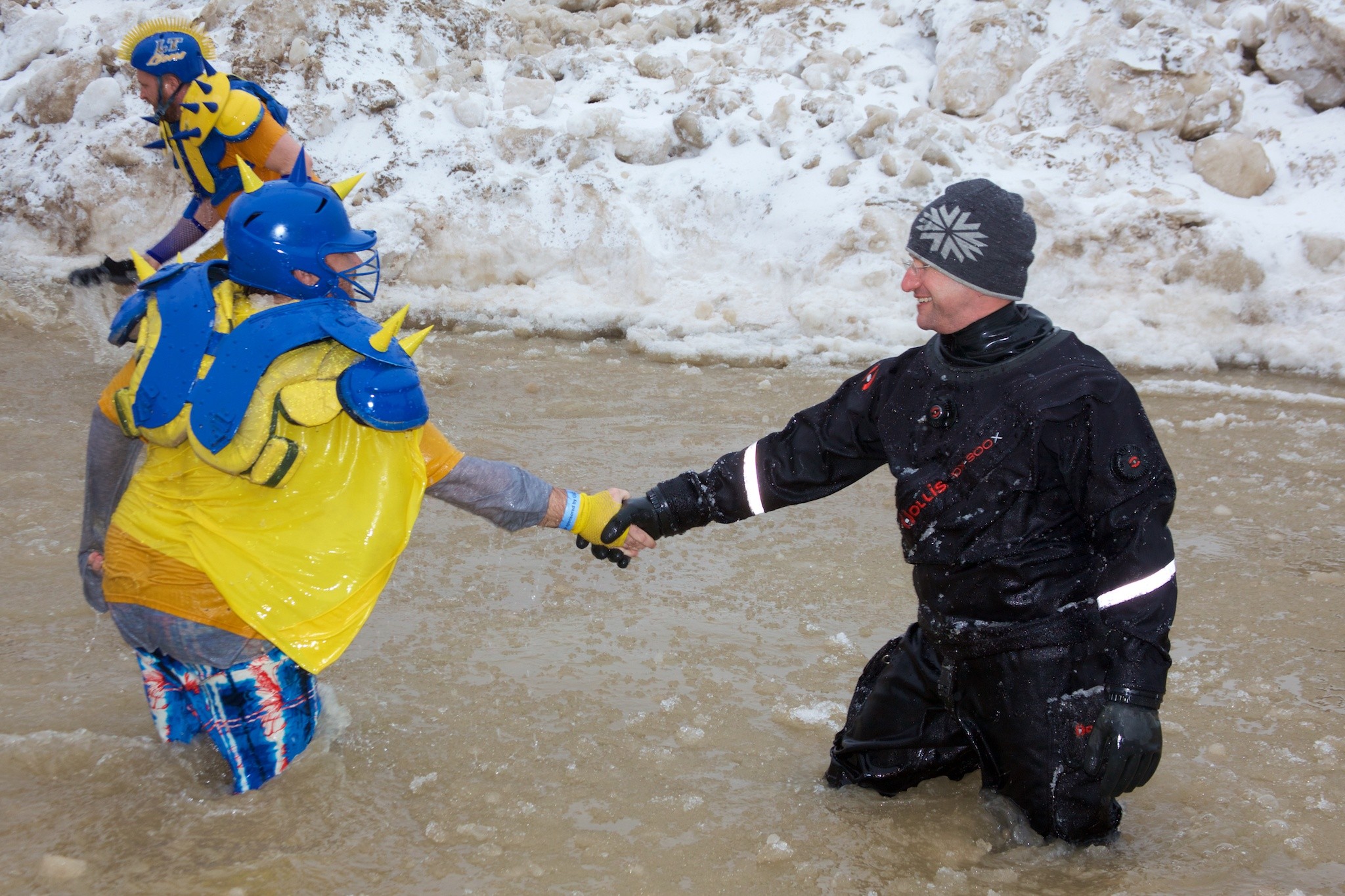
[909,178,1038,300]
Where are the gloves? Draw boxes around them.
[576,496,663,568]
[68,256,138,288]
[1084,700,1163,799]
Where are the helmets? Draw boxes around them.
[116,18,217,85]
[222,141,379,305]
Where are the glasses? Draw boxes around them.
[900,258,933,274]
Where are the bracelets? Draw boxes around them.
[557,489,579,531]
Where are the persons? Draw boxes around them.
[576,178,1176,849]
[80,144,655,795]
[67,15,314,283]
